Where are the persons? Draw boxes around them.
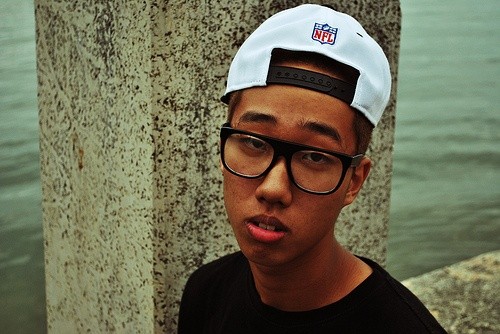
[175,3,448,334]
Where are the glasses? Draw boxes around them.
[219,122,364,195]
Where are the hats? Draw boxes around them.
[221,4,392,128]
